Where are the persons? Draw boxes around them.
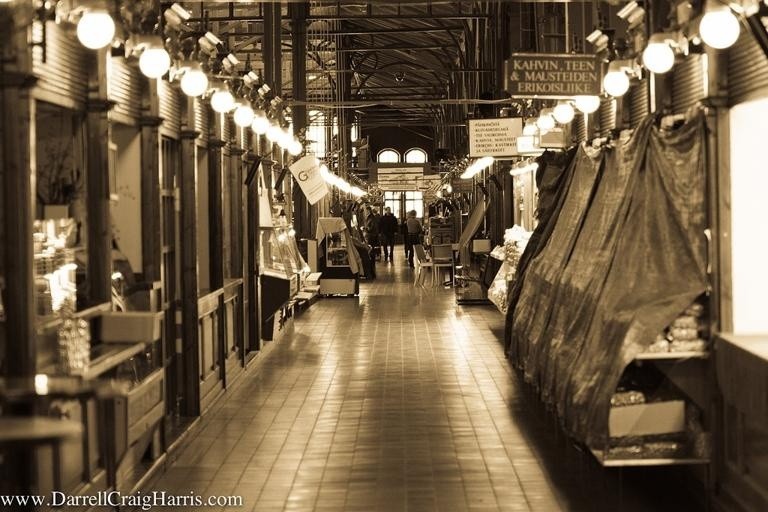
[378,207,398,266]
[401,212,414,260]
[360,207,380,264]
[372,208,381,262]
[406,210,423,271]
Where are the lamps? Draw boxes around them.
[76,0,364,199]
[459,0,741,181]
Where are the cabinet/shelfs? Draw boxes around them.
[591,351,714,470]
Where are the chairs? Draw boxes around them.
[430,245,455,288]
[413,245,433,287]
